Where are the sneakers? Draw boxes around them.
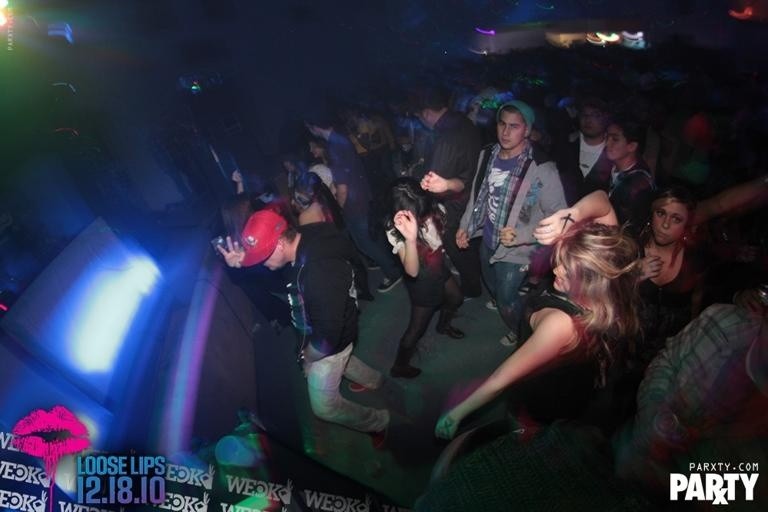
[377,275,401,294]
[499,331,518,347]
[373,428,386,449]
[349,383,367,392]
[486,300,498,310]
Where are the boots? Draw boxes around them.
[390,348,421,379]
[436,311,464,339]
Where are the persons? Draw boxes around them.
[216,34,768,511]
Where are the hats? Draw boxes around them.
[497,99,535,137]
[239,209,288,266]
